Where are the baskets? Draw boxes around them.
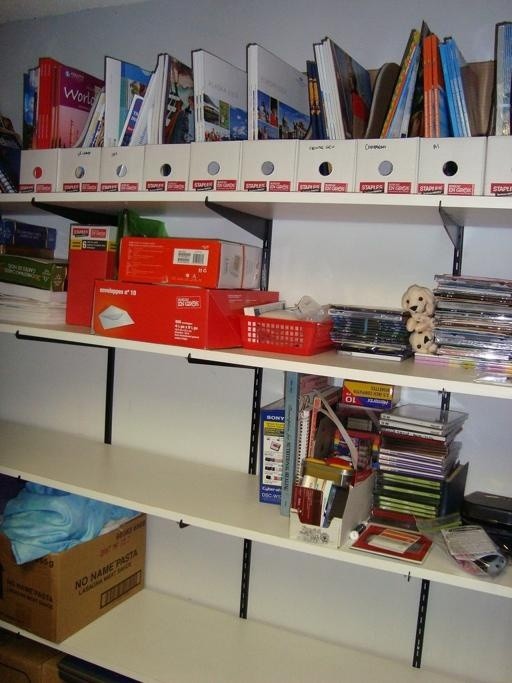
[236,311,334,357]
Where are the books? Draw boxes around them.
[0,16,512,193]
[0,277,68,325]
[294,380,470,568]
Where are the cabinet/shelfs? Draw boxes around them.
[1,187,511,682]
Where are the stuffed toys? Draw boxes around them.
[402,285,434,354]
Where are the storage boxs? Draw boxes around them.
[0,472,147,646]
